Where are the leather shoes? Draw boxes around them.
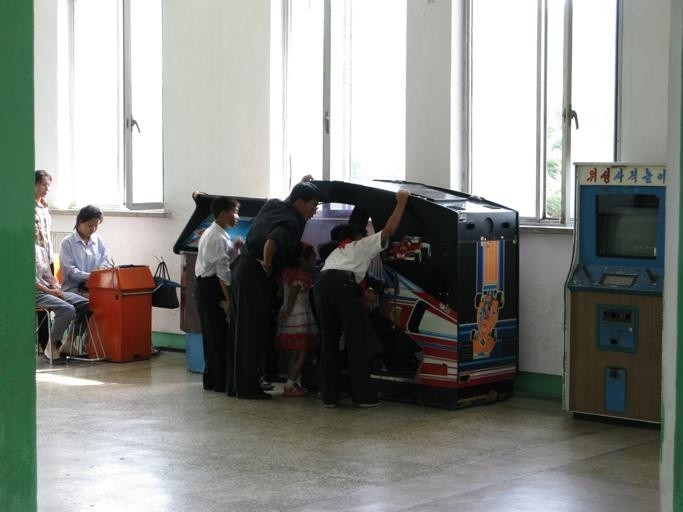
[214,386,226,392]
[237,390,270,400]
[43,352,64,364]
[61,351,88,358]
[203,384,214,389]
[226,389,237,395]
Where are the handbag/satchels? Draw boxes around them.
[152,263,181,310]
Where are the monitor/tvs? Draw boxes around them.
[595,194,659,258]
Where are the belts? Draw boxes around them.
[318,270,353,276]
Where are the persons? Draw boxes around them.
[308,242,349,398]
[272,241,320,397]
[313,189,410,409]
[59,206,159,357]
[196,197,245,392]
[35,170,54,354]
[225,175,321,399]
[34,211,89,364]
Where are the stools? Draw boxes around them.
[36,304,108,376]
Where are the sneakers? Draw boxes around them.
[293,386,308,393]
[284,388,303,397]
[324,399,338,407]
[356,398,383,407]
